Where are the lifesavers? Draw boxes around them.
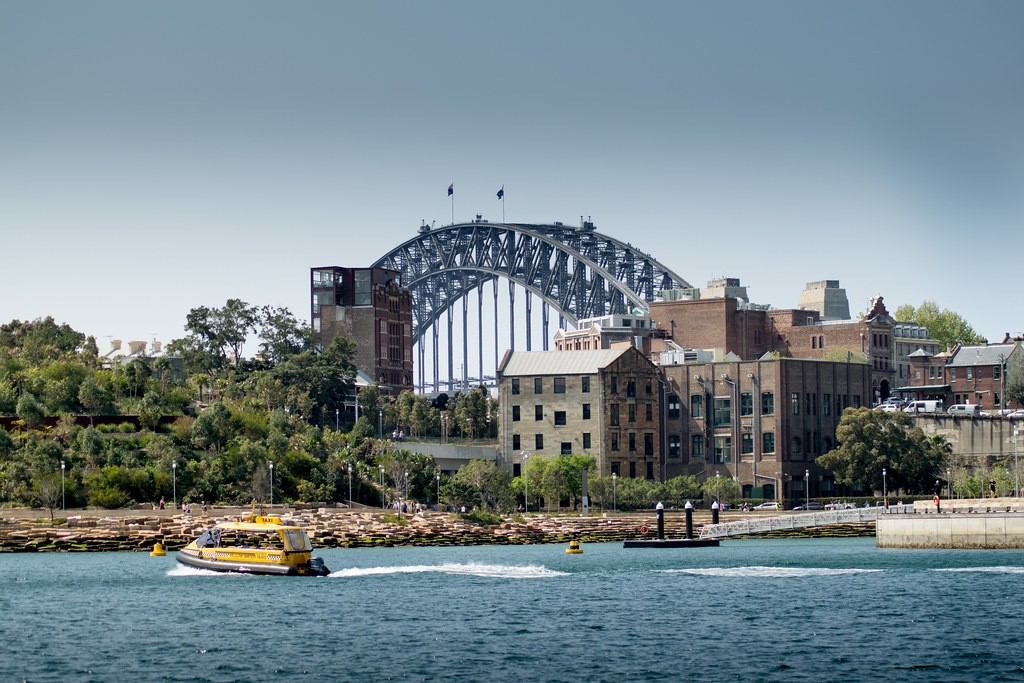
[641,526,648,534]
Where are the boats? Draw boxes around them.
[176,514,330,577]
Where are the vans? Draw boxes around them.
[904,400,980,415]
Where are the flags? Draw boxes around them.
[448,184,453,196]
[497,186,504,199]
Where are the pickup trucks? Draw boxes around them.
[825,500,856,510]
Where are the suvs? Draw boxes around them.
[874,405,901,413]
[753,502,784,510]
[882,397,906,406]
[794,503,825,510]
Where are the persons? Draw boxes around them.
[395,498,468,513]
[933,480,941,499]
[239,539,262,550]
[159,496,209,514]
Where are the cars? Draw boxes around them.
[980,410,1024,418]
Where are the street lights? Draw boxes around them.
[715,471,719,501]
[947,470,951,500]
[348,464,352,509]
[336,409,339,429]
[883,469,887,507]
[405,471,408,500]
[61,460,66,509]
[522,451,529,511]
[437,475,440,505]
[172,461,176,512]
[806,471,809,511]
[441,414,448,441]
[379,466,384,509]
[355,400,359,423]
[269,461,273,508]
[379,411,382,439]
[612,474,617,512]
